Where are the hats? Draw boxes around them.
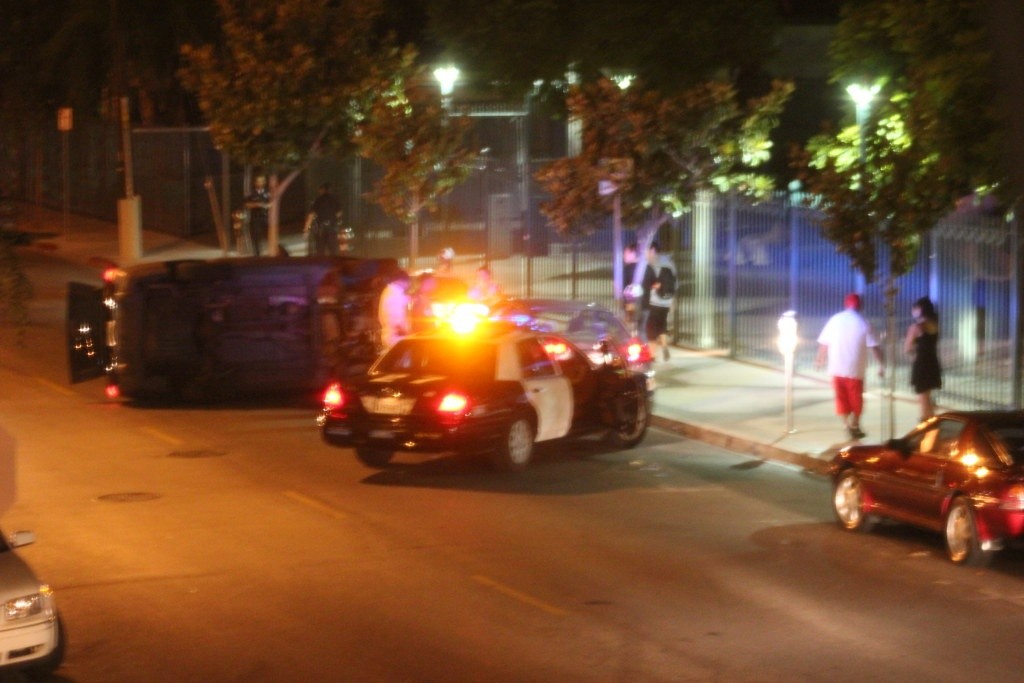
[845,294,859,307]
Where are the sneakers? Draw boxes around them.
[848,426,867,440]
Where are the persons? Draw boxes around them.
[620,241,678,364]
[902,296,944,424]
[812,289,886,437]
[376,264,503,354]
[242,170,273,259]
[301,180,343,261]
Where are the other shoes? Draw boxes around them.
[663,346,672,362]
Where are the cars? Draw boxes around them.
[831,403,1023,570]
[327,331,655,477]
[476,301,663,383]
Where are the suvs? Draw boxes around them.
[67,248,399,412]
